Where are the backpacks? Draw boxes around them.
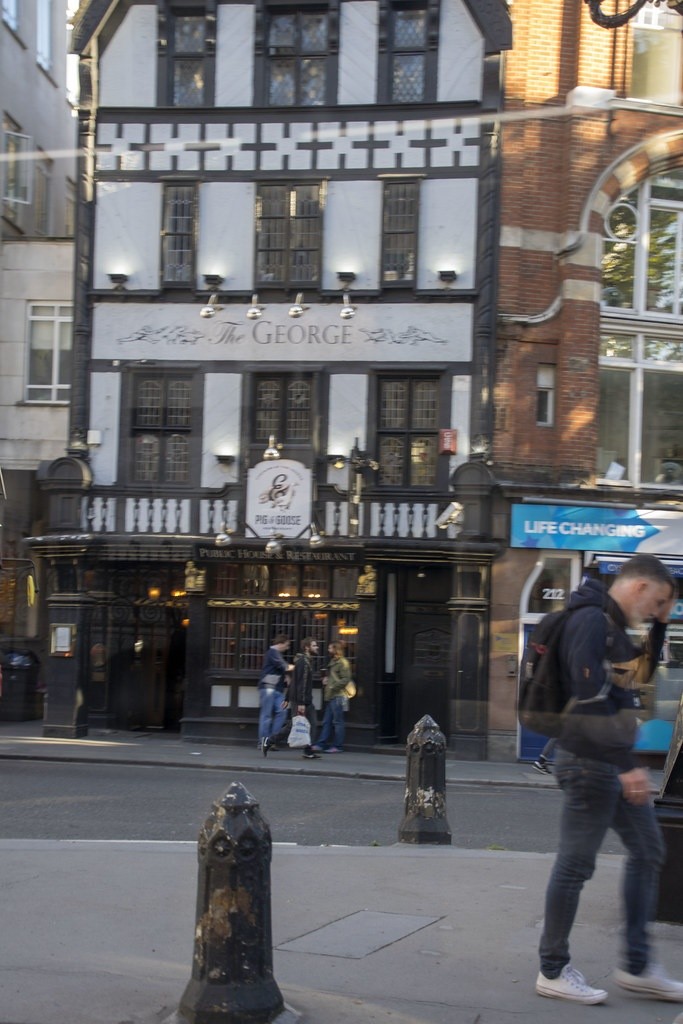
[518,604,649,739]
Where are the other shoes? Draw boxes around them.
[262,737,271,757]
[531,761,552,775]
[311,745,323,750]
[323,747,343,753]
[301,751,320,759]
[257,739,280,751]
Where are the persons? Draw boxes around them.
[260,636,323,759]
[532,737,559,775]
[312,640,350,753]
[258,634,290,751]
[536,553,683,1002]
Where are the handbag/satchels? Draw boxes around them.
[343,682,356,698]
[287,712,311,748]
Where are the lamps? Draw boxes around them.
[266,526,280,554]
[340,295,355,319]
[471,450,485,458]
[336,272,355,281]
[107,273,127,282]
[263,436,282,461]
[214,517,232,547]
[439,270,456,282]
[200,295,219,318]
[202,274,224,284]
[434,503,464,531]
[87,429,102,446]
[246,294,264,320]
[332,455,352,472]
[215,454,234,465]
[295,522,325,549]
[289,293,305,318]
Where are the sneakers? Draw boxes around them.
[613,962,683,1001]
[536,964,609,1004]
